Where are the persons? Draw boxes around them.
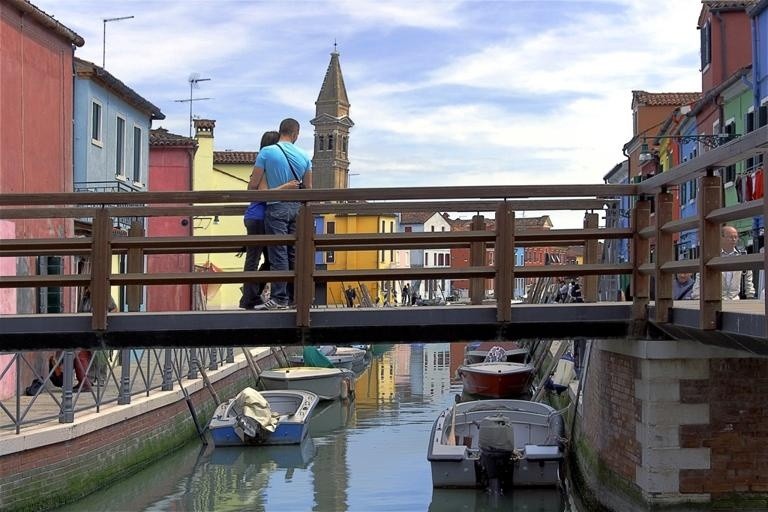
[345,285,353,307]
[692,225,755,300]
[248,118,313,311]
[407,283,411,303]
[556,279,583,303]
[239,130,300,310]
[672,258,696,299]
[402,285,408,303]
[72,286,118,392]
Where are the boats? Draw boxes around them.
[208,429,316,480]
[207,387,322,448]
[308,397,355,434]
[426,485,564,511]
[462,343,529,363]
[256,366,359,402]
[454,361,535,399]
[368,343,395,356]
[422,398,562,489]
[290,347,368,372]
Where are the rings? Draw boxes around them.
[295,185,297,187]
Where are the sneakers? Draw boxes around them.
[254,299,288,310]
[239,296,265,308]
